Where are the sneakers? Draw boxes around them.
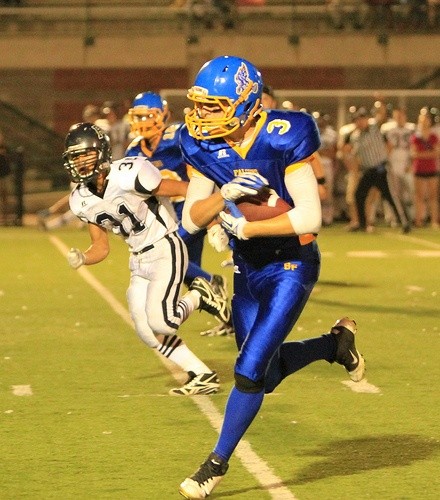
[190,276,233,326]
[169,370,221,396]
[332,317,366,382]
[199,325,235,338]
[177,454,229,500]
[211,275,224,286]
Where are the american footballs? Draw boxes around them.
[225,187,294,223]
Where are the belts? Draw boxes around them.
[133,244,155,256]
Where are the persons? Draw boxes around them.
[62,122,233,396]
[176,56,366,500]
[125,91,233,335]
[261,84,328,201]
[337,100,440,232]
[281,101,338,227]
[329,0,440,33]
[172,0,240,44]
[38,101,125,228]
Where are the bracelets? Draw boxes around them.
[315,176,326,184]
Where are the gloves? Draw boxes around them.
[205,223,229,252]
[218,200,248,240]
[220,172,269,202]
[67,248,85,269]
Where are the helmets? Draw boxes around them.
[184,56,263,140]
[127,91,170,139]
[61,121,111,183]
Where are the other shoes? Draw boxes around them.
[404,226,410,233]
[350,225,366,232]
